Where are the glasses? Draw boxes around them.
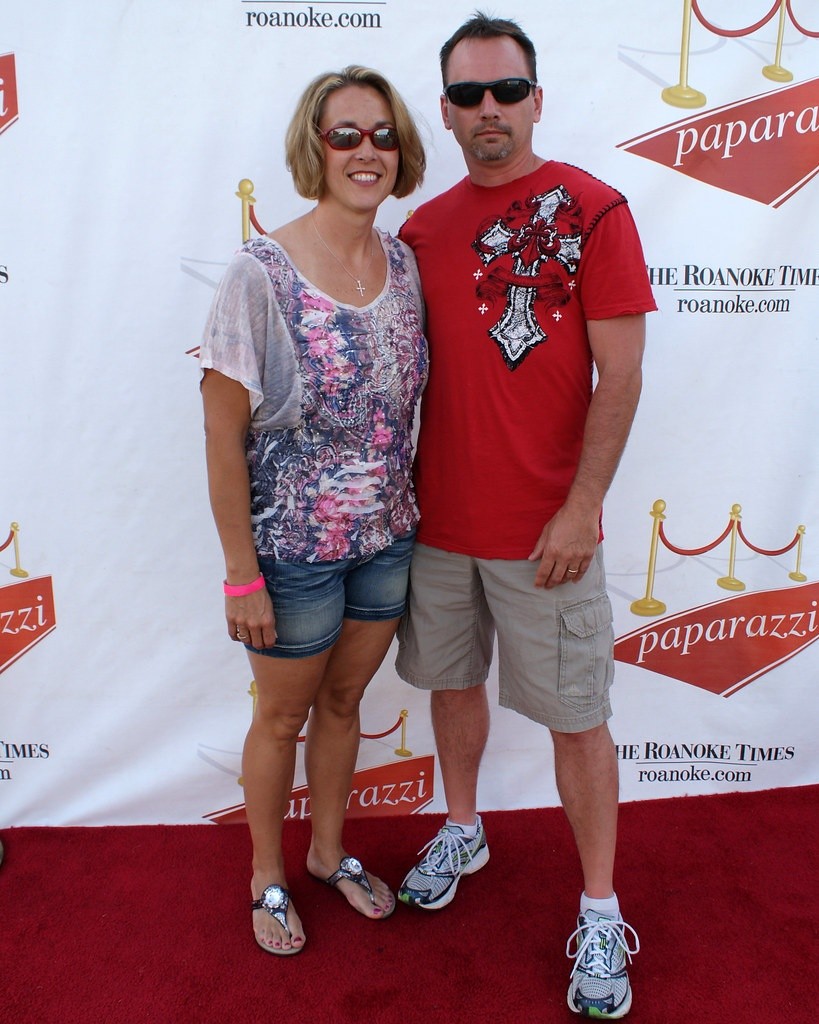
[317,124,400,152]
[444,76,539,108]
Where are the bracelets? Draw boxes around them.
[223,573,265,596]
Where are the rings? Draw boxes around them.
[568,569,578,573]
[237,633,247,640]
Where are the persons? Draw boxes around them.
[198,64,429,954]
[397,10,659,1020]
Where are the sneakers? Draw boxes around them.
[566,910,633,1022]
[397,815,490,909]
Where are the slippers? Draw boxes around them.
[309,851,399,924]
[249,882,306,959]
